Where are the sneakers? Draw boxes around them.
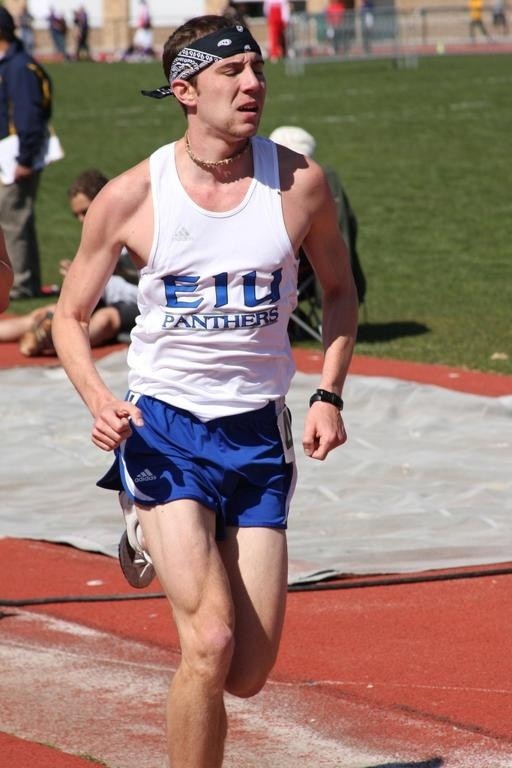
[115,484,160,589]
[19,308,55,358]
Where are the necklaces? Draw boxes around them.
[181,127,253,171]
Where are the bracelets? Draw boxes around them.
[308,386,344,411]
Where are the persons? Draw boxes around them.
[263,122,368,345]
[0,3,55,301]
[48,8,365,767]
[48,11,70,60]
[490,1,510,37]
[17,4,38,59]
[262,1,294,59]
[71,6,95,62]
[358,0,375,53]
[324,0,347,54]
[0,168,144,355]
[465,0,495,46]
[0,220,17,318]
[224,0,249,27]
[121,0,158,61]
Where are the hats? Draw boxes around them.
[268,122,318,160]
